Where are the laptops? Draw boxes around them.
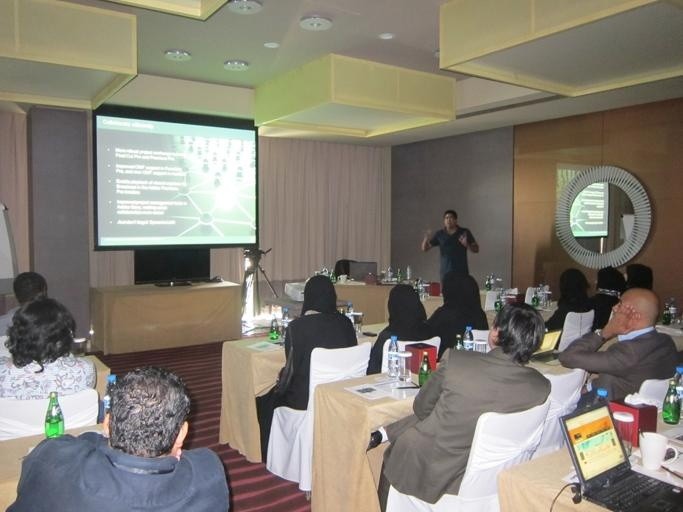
[560,403,683,511]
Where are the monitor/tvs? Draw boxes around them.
[134,246,210,287]
[349,261,377,281]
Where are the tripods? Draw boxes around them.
[250,265,279,317]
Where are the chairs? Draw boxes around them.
[265,329,495,505]
[485,285,554,311]
[382,368,679,512]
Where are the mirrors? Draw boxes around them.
[554,162,652,270]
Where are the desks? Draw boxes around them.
[0,353,114,511]
[321,278,503,331]
[86,275,244,358]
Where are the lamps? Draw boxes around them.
[102,0,231,23]
[0,0,138,113]
[254,49,456,140]
[439,0,681,97]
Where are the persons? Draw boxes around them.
[366,283,447,374]
[365,300,553,512]
[541,268,600,331]
[0,271,49,336]
[559,288,678,401]
[624,263,654,295]
[589,266,627,330]
[3,365,229,512]
[255,275,357,463]
[0,298,97,400]
[422,208,480,283]
[427,271,489,347]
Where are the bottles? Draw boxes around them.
[103,375,117,437]
[413,279,430,298]
[454,333,466,350]
[312,266,336,283]
[340,302,363,333]
[281,307,290,340]
[613,411,635,458]
[674,367,683,418]
[44,391,68,438]
[388,336,400,379]
[269,314,280,340]
[661,295,678,325]
[662,376,680,424]
[419,352,431,387]
[484,272,503,291]
[463,326,474,351]
[494,285,553,309]
[591,387,608,407]
[379,263,410,283]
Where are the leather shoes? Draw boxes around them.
[366,431,383,451]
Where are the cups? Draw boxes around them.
[337,274,347,281]
[399,350,413,382]
[637,432,680,472]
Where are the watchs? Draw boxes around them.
[594,329,602,336]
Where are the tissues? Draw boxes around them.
[423,281,440,296]
[608,391,661,448]
[404,342,437,374]
[500,286,525,305]
